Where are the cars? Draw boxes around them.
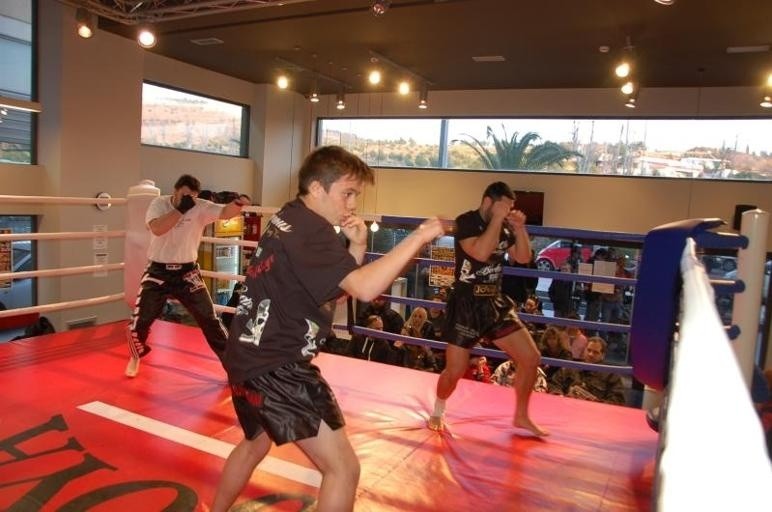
[0,240,33,310]
[706,255,771,300]
[535,238,593,271]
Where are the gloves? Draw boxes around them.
[234,194,251,207]
[175,194,196,215]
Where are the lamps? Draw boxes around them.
[417,84,429,110]
[76,8,160,47]
[308,78,349,112]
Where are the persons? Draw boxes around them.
[458,247,626,406]
[220,284,242,328]
[323,291,448,373]
[211,147,443,512]
[126,175,251,377]
[428,182,551,437]
